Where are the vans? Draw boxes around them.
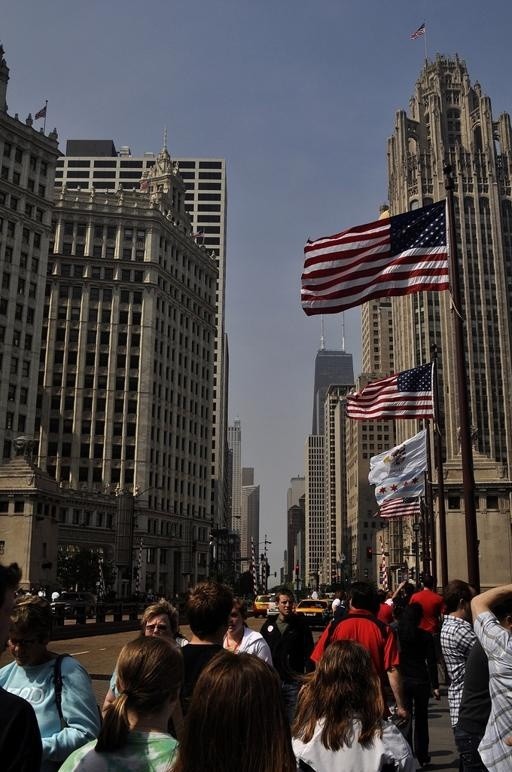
[48,590,94,617]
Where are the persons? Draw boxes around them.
[1,596,104,770]
[58,573,512,772]
[0,564,46,771]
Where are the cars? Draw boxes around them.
[295,598,330,624]
[253,595,270,618]
[267,592,280,616]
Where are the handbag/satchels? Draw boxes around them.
[54,653,103,729]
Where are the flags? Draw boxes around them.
[368,428,430,518]
[302,198,451,317]
[346,362,435,421]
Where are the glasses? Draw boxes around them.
[145,624,167,631]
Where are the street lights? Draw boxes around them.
[128,483,163,583]
[212,513,243,580]
